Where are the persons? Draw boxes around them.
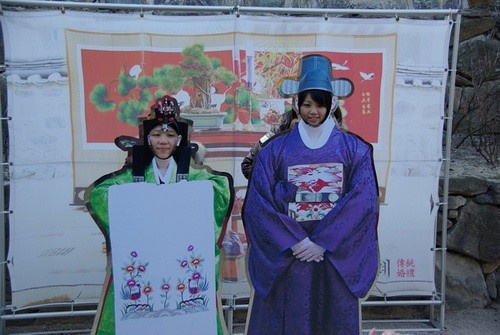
[90,95,231,335]
[241,54,381,335]
